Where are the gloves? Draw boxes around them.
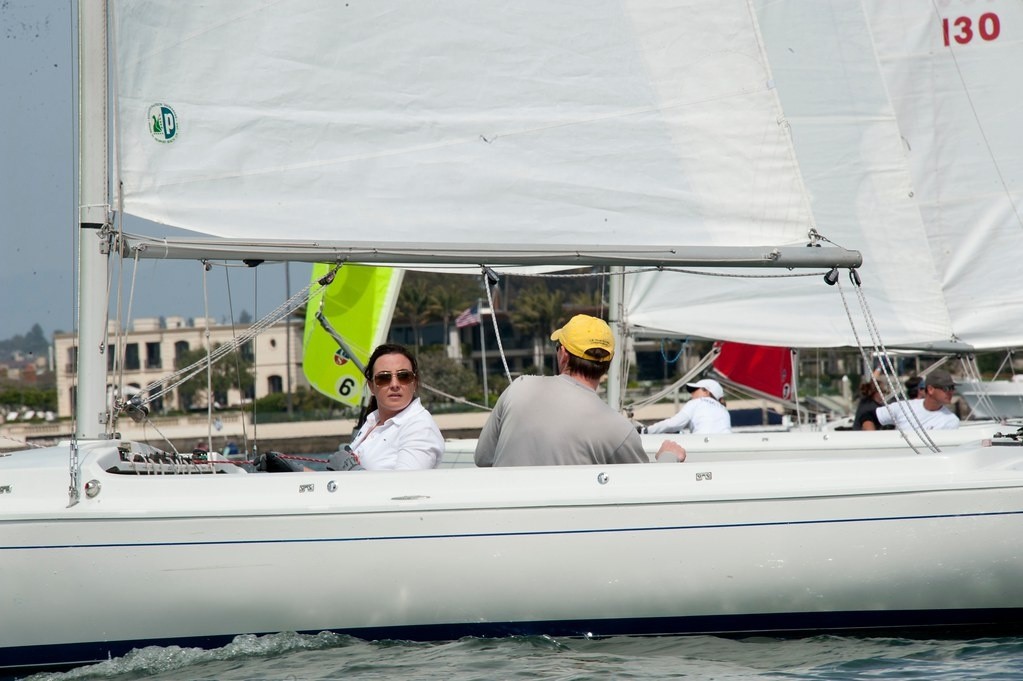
[254,451,304,472]
[325,450,365,471]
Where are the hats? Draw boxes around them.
[687,379,724,400]
[908,380,927,392]
[925,369,962,387]
[551,314,615,362]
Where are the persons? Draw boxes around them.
[474,313,687,467]
[254,345,444,473]
[635,378,731,434]
[852,371,971,432]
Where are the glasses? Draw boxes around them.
[372,371,416,385]
[932,384,955,393]
[555,342,562,352]
[877,389,887,395]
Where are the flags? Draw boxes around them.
[455,305,480,327]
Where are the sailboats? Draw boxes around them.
[2,0,1022,676]
